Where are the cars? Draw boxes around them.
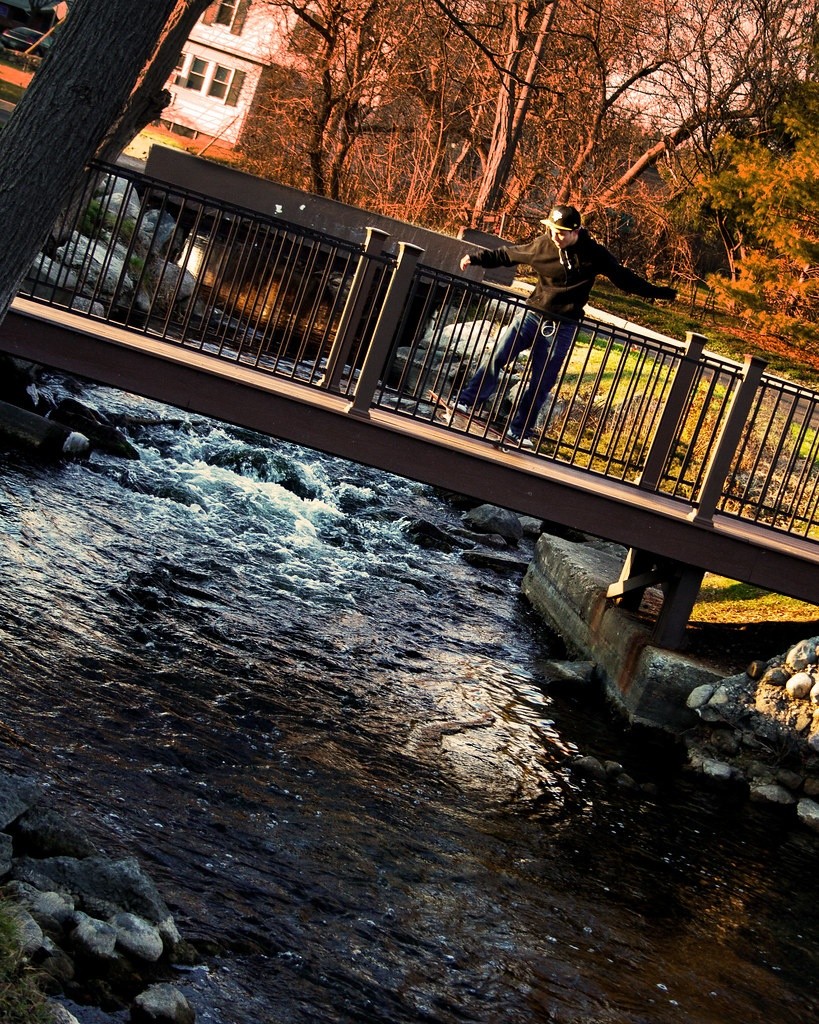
[0,25,53,58]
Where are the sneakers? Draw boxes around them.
[453,399,467,413]
[507,427,533,448]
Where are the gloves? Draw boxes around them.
[655,286,678,300]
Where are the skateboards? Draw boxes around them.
[428,389,524,452]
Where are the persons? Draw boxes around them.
[452,204,680,448]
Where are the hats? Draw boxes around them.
[540,205,580,231]
